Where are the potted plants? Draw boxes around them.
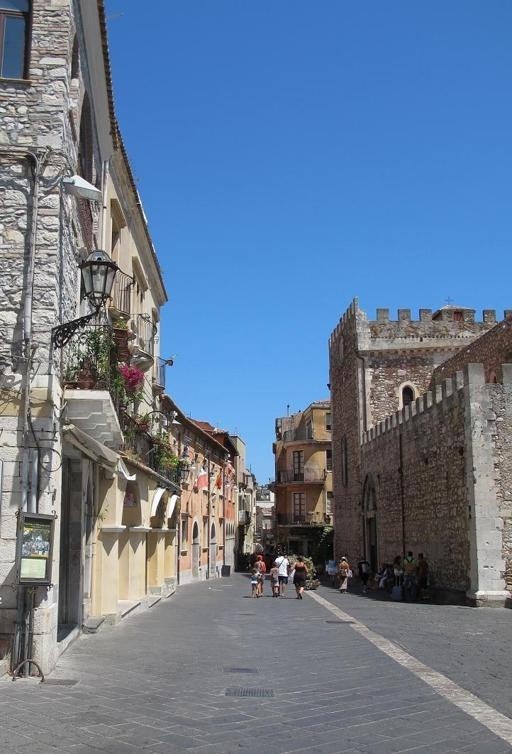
[138,413,152,431]
[114,319,135,360]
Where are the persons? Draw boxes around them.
[238,549,291,598]
[289,557,308,599]
[325,551,429,602]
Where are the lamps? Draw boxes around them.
[52,234,118,347]
[63,175,102,202]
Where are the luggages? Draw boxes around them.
[391,575,403,602]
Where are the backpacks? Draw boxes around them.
[405,558,416,576]
